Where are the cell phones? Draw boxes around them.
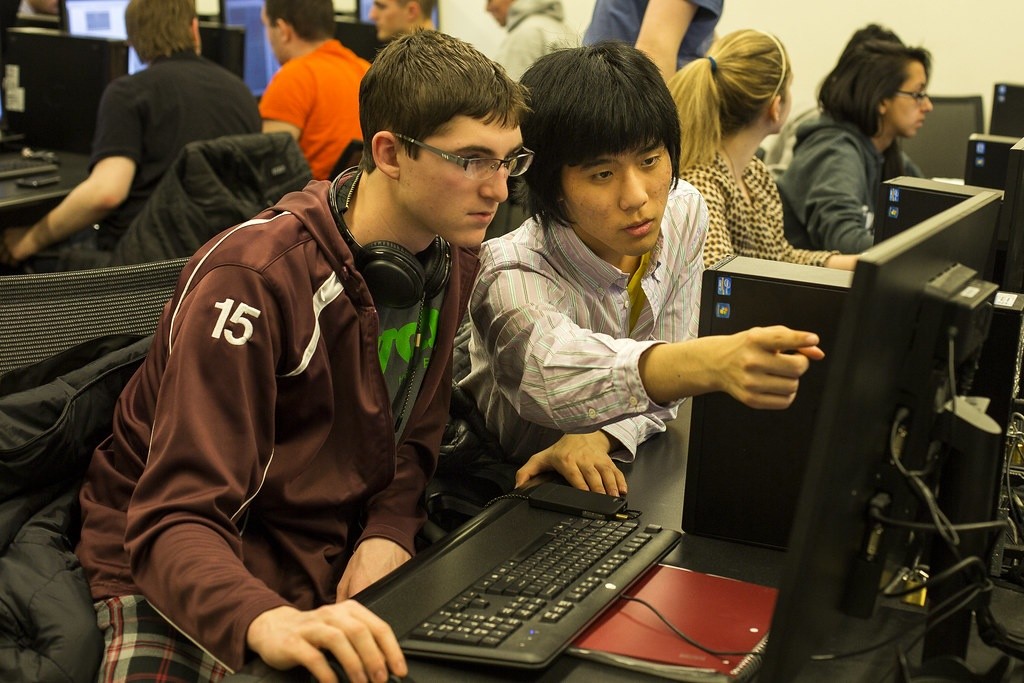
[529,485,629,522]
[15,175,61,187]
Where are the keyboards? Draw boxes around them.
[392,522,687,674]
[0,158,59,180]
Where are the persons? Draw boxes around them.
[76,28,535,683]
[780,24,934,254]
[0,0,262,276]
[581,0,725,84]
[369,0,436,40]
[664,29,859,271]
[485,0,581,85]
[467,40,824,498]
[257,0,372,180]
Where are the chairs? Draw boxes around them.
[113,129,310,269]
[1,254,192,682]
[895,93,987,181]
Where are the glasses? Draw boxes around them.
[890,89,930,104]
[389,133,534,181]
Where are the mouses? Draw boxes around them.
[294,651,415,683]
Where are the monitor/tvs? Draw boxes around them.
[763,191,1024,683]
[1002,139,1024,294]
[58,0,149,76]
[220,0,283,101]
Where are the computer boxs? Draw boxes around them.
[4,26,130,154]
[198,20,246,82]
[681,85,1024,575]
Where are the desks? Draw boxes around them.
[0,145,88,207]
[220,402,785,683]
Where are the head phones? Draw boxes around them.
[328,165,452,309]
